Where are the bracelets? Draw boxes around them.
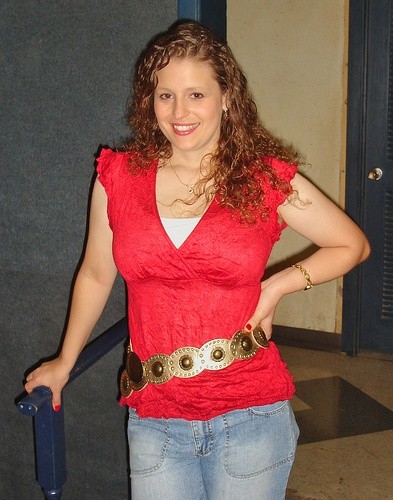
[290,265,313,290]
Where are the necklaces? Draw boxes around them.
[168,157,211,193]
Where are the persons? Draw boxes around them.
[23,24,372,500]
